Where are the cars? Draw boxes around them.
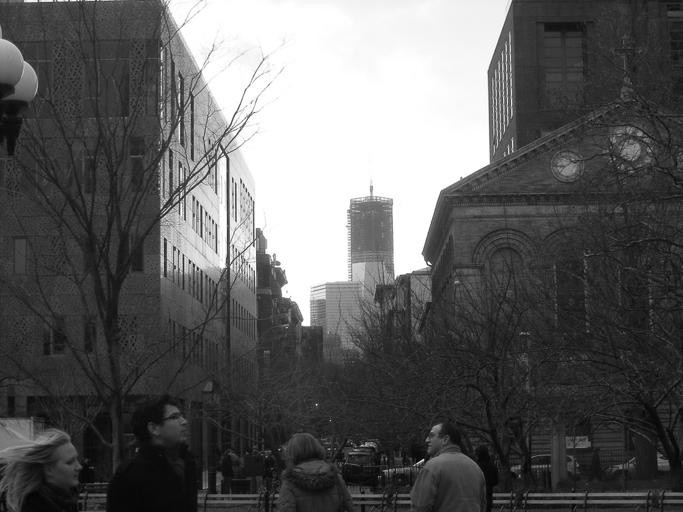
[510,453,582,482]
[383,457,425,486]
[321,436,382,484]
[251,448,283,462]
[607,452,670,478]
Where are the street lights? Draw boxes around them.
[202,381,222,494]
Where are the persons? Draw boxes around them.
[410,422,498,512]
[106,393,203,511]
[0,428,83,512]
[276,432,353,512]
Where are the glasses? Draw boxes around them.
[162,412,186,420]
